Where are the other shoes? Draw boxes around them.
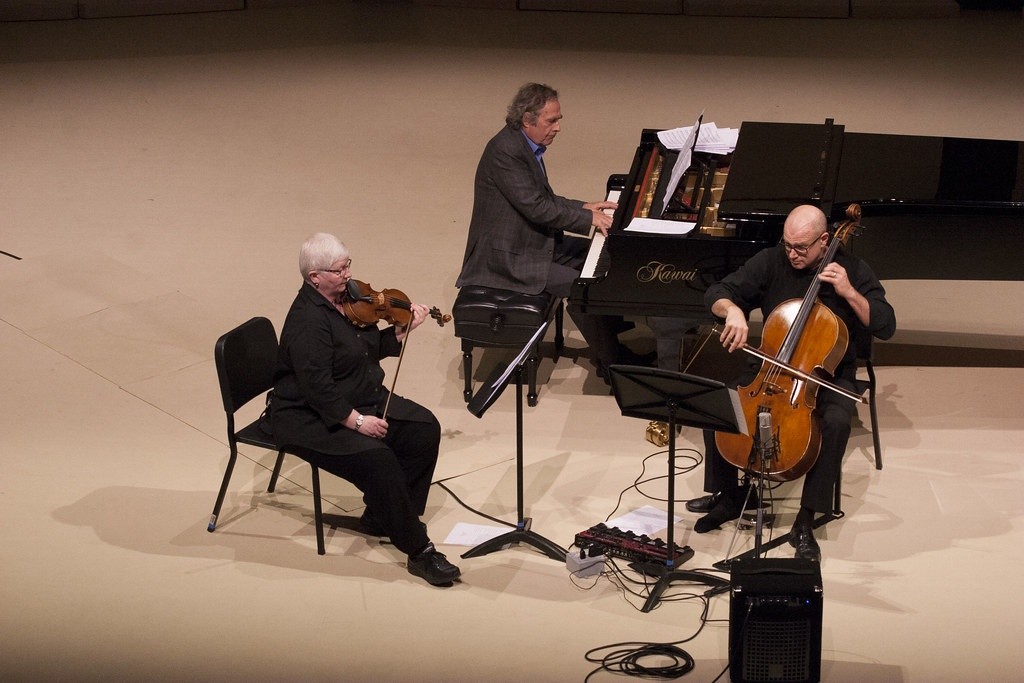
[407,542,461,586]
[358,505,428,537]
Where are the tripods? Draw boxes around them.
[460,318,569,563]
[609,365,740,613]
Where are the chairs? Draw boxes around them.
[207,317,325,557]
[829,322,882,515]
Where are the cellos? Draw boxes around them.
[714,201,868,484]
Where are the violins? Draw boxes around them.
[341,279,452,330]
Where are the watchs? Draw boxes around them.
[354,413,364,431]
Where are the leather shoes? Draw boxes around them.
[686,490,743,512]
[789,522,822,564]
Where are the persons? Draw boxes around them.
[265,232,461,585]
[685,205,896,563]
[455,81,659,384]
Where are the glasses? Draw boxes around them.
[777,236,821,255]
[317,259,352,277]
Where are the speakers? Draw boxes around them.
[728,558,823,683]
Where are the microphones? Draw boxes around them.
[758,413,774,472]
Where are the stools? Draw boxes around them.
[451,285,565,407]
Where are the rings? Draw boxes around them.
[832,272,837,278]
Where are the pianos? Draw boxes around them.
[564,109,852,381]
